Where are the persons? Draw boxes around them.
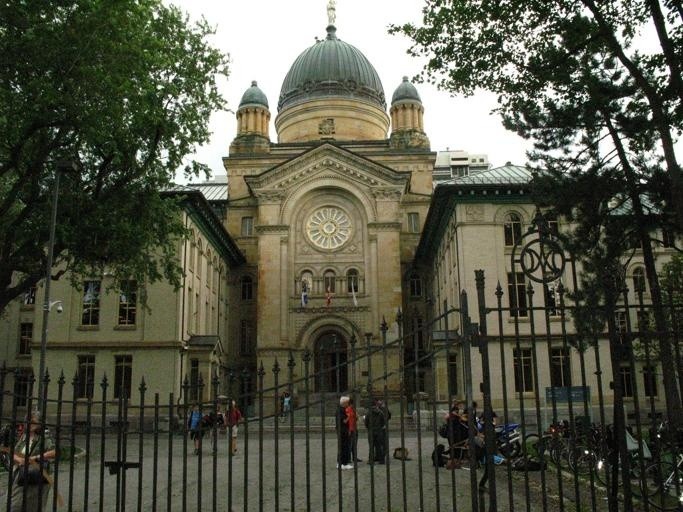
[444,398,499,470]
[335,395,353,470]
[346,397,362,463]
[280,389,292,422]
[4,410,57,511]
[374,398,390,461]
[186,398,244,456]
[365,402,386,464]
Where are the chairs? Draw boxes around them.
[609,424,652,458]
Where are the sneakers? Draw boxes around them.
[232,449,237,456]
[336,458,386,470]
[209,449,213,456]
[193,448,198,455]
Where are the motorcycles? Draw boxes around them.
[473,418,521,464]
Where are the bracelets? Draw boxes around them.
[33,455,38,462]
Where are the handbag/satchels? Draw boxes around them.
[446,458,460,470]
[17,464,48,487]
[393,448,409,460]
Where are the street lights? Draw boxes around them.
[38,300,63,424]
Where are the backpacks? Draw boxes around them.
[438,425,448,439]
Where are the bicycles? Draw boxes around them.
[536,421,683,510]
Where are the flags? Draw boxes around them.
[350,276,358,306]
[301,282,308,307]
[327,278,332,306]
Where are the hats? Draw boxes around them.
[454,400,463,405]
